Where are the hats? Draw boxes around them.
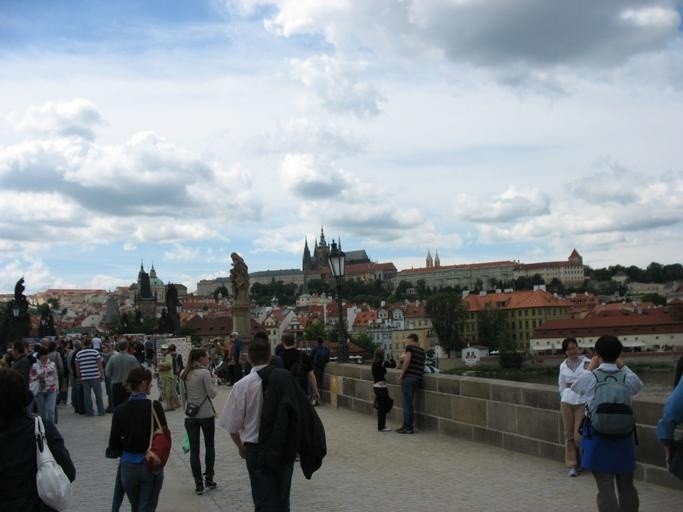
[161,344,169,350]
[230,332,239,336]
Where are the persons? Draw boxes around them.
[157,344,182,412]
[571,334,644,512]
[180,348,221,495]
[99,343,117,413]
[559,337,595,476]
[371,347,395,431]
[75,337,105,415]
[27,334,69,351]
[0,367,76,512]
[113,332,135,346]
[655,354,683,483]
[0,341,11,368]
[395,334,425,434]
[169,344,185,394]
[227,333,233,386]
[253,331,284,370]
[145,337,155,367]
[138,340,146,362]
[105,338,139,413]
[218,339,327,512]
[106,365,171,512]
[283,334,321,403]
[231,332,242,386]
[69,332,112,351]
[72,340,83,414]
[10,342,33,376]
[145,336,154,367]
[45,343,64,405]
[309,337,330,405]
[233,331,243,385]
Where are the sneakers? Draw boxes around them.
[568,468,579,477]
[195,487,206,496]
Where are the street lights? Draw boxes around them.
[327,243,349,363]
[176,303,182,327]
[11,305,24,339]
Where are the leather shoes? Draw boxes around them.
[205,479,218,489]
[396,428,415,435]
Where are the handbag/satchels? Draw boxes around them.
[179,375,209,418]
[144,400,171,475]
[34,415,73,511]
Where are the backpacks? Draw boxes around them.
[586,369,639,447]
[173,353,180,373]
